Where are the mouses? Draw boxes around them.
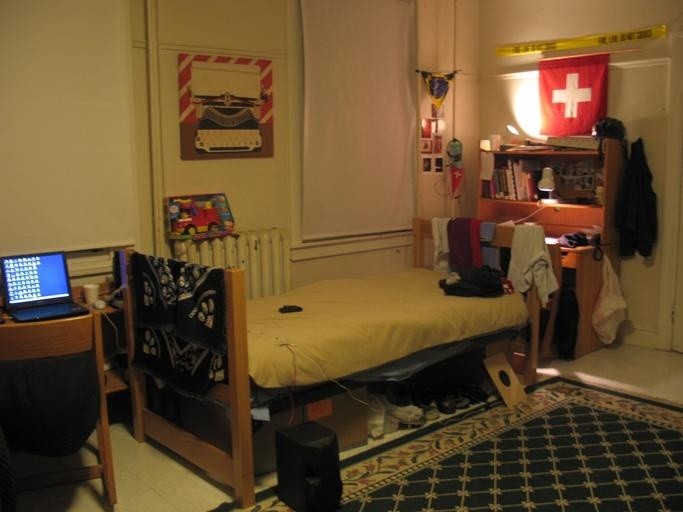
[93,299,107,309]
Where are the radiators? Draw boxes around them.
[172,227,290,304]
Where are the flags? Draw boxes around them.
[538,53,609,136]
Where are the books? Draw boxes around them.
[481,157,542,201]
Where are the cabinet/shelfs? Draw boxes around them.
[1,283,128,396]
[477,138,626,358]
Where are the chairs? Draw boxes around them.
[1,309,116,509]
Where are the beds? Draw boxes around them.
[117,216,542,512]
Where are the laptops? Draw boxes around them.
[0,251,90,323]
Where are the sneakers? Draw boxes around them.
[368,384,483,427]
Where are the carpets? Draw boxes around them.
[214,374,683,511]
